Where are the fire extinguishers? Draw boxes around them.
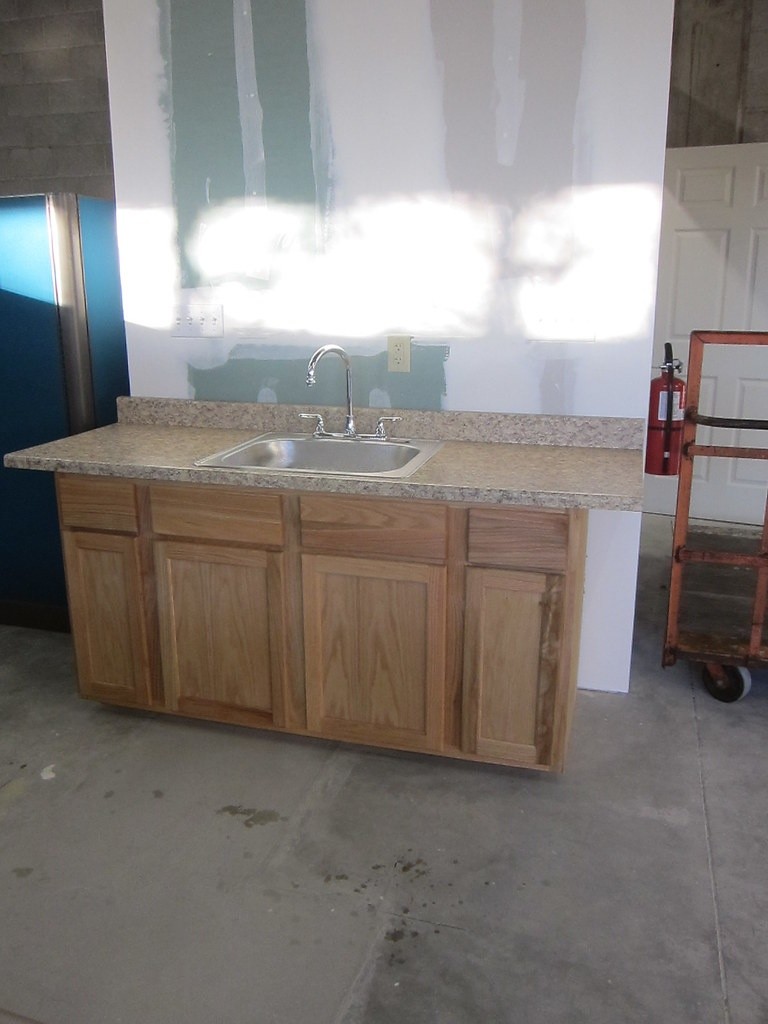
[644,343,686,476]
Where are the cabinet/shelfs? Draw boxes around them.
[53,471,588,775]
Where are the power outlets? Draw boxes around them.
[388,334,411,373]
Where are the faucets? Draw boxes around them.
[305,343,358,439]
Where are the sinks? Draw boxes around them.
[194,434,443,478]
